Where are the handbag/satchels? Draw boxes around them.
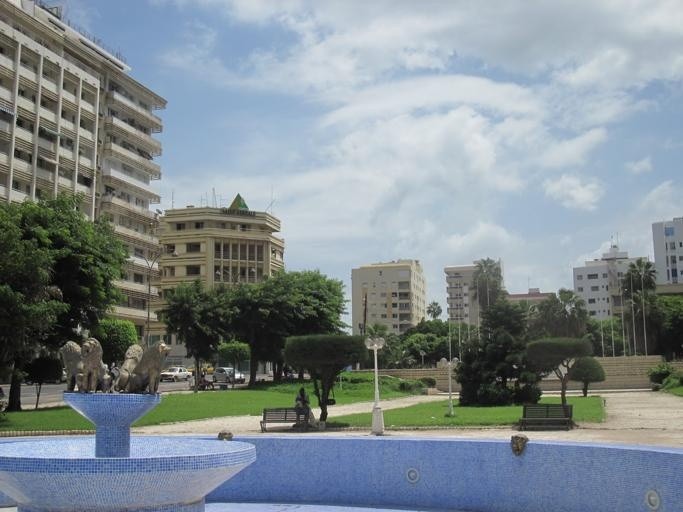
[327,399,335,405]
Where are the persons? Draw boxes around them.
[199,374,210,391]
[207,365,213,374]
[295,387,308,418]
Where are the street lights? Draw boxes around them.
[440,358,458,416]
[126,249,179,345]
[365,338,386,434]
[419,351,425,367]
[216,267,255,389]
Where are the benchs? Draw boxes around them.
[259,408,309,432]
[519,404,572,431]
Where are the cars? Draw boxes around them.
[187,364,213,376]
[162,366,190,382]
[212,367,245,384]
